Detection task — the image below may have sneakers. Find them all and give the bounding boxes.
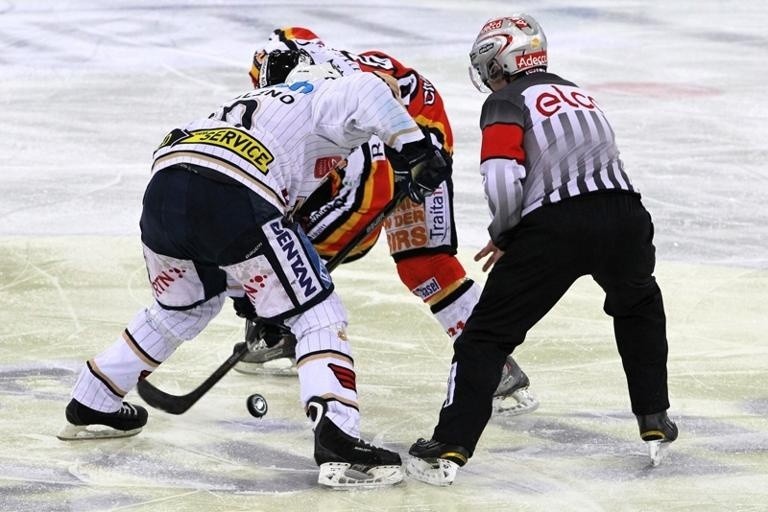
[408,436,471,468]
[233,332,297,364]
[494,355,530,398]
[636,409,679,443]
[313,415,402,473]
[64,398,148,431]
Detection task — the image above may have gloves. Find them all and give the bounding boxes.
[399,138,453,206]
[229,292,258,321]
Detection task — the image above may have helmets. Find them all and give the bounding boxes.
[259,48,311,89]
[467,11,548,93]
[285,61,344,84]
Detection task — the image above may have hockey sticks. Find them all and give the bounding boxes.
[137,185,410,413]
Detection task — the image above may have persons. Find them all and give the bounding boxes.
[235,27,529,397]
[66,52,450,469]
[403,12,677,468]
